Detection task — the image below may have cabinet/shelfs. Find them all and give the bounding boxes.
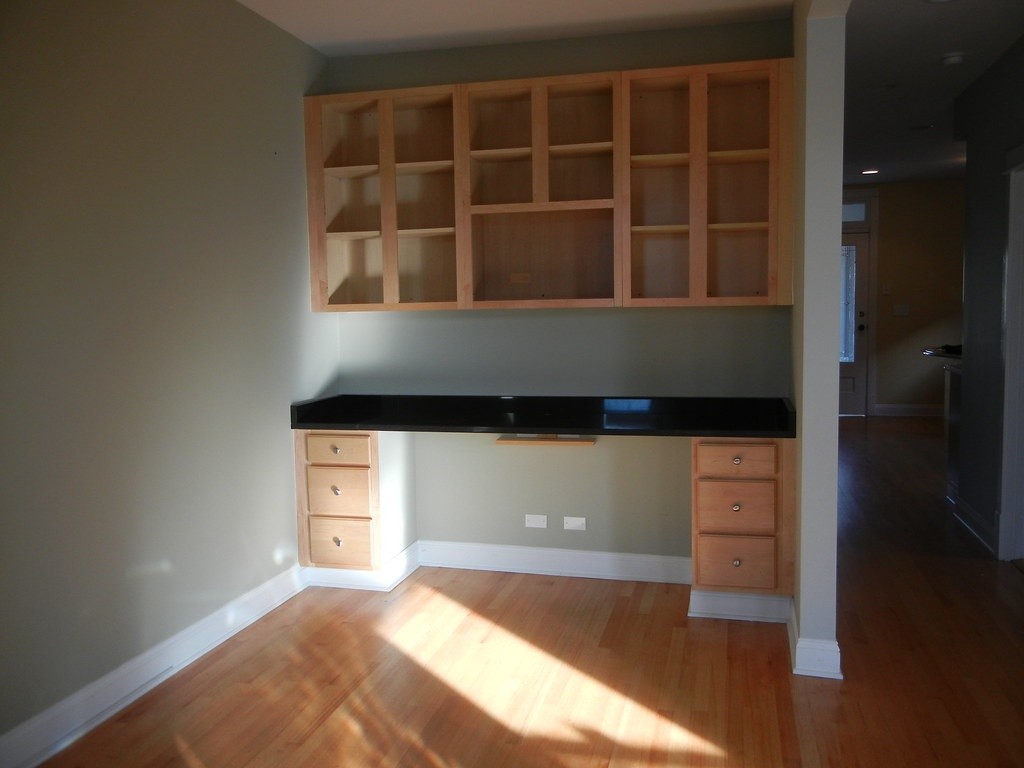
[303,61,793,313]
[293,397,794,620]
